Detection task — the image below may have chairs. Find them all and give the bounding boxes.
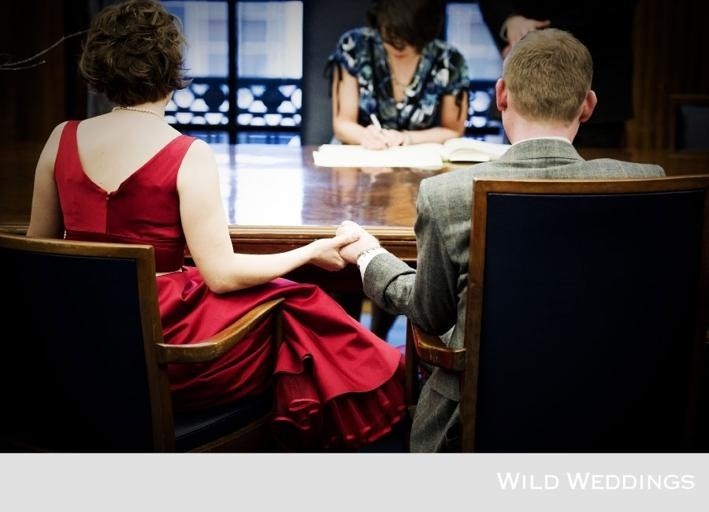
[403,173,708,453]
[0,232,285,453]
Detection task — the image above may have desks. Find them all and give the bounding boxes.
[0,139,709,263]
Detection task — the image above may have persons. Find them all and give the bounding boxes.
[24,0,670,453]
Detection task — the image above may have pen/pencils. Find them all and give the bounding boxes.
[369,113,390,149]
